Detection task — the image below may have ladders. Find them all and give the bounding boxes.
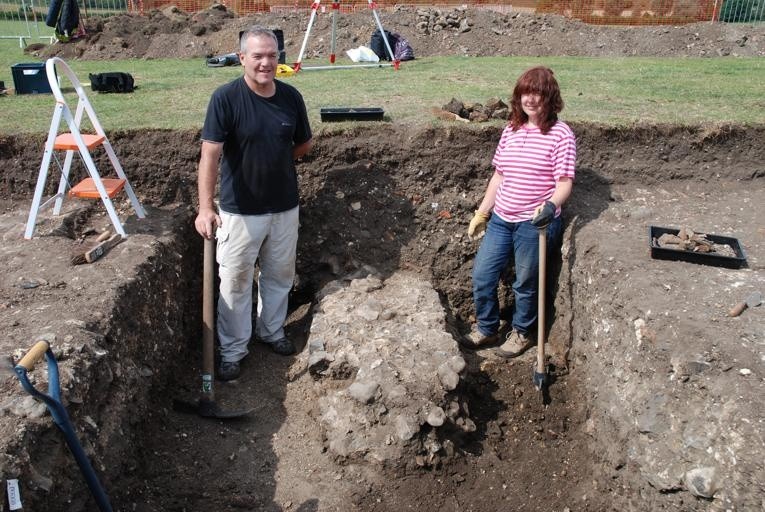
[24,57,147,241]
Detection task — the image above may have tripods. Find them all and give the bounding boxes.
[290,0,401,75]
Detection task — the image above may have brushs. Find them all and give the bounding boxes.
[70,233,121,266]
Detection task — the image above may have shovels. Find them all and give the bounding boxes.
[730,292,762,317]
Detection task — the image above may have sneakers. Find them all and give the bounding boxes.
[499,327,534,358]
[217,361,241,380]
[462,329,498,350]
[270,337,295,355]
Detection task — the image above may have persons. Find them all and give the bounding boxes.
[460,67,576,355]
[193,27,313,381]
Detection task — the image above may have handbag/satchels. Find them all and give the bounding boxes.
[395,37,413,61]
[89,73,134,93]
[371,30,396,60]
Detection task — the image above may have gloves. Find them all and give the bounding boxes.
[468,209,490,242]
[531,201,556,229]
